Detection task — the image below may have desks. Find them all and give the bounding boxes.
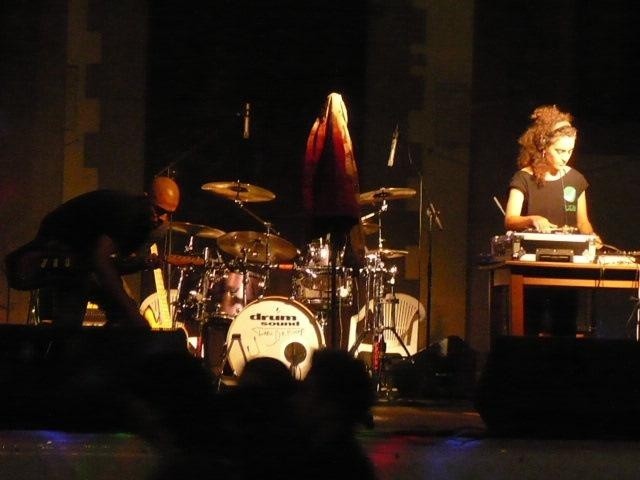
[479,261,640,355]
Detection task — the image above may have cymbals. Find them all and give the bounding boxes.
[165,222,226,240]
[366,249,408,262]
[165,254,204,267]
[201,179,277,203]
[218,231,296,263]
[357,222,380,234]
[356,186,416,205]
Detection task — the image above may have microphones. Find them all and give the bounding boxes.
[386,124,399,168]
[241,102,252,141]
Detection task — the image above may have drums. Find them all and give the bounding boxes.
[226,294,325,385]
[292,266,354,310]
[200,262,271,330]
[294,233,347,275]
[173,264,210,325]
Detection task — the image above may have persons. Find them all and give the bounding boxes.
[503,102,605,339]
[34,175,182,331]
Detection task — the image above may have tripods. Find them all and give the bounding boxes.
[345,325,416,396]
